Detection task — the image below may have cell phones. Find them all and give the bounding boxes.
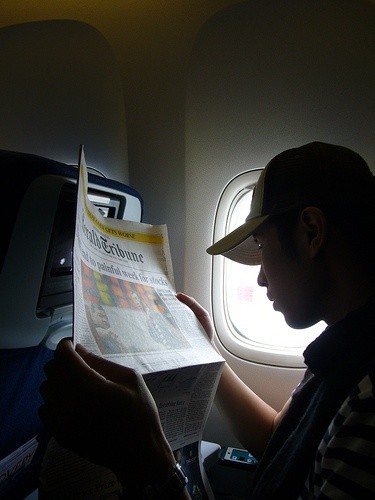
[223,446,258,468]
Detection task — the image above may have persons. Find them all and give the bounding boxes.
[37,141,375,500]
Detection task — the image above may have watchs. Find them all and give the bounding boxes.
[115,461,189,498]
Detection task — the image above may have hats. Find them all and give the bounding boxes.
[207,141,375,277]
